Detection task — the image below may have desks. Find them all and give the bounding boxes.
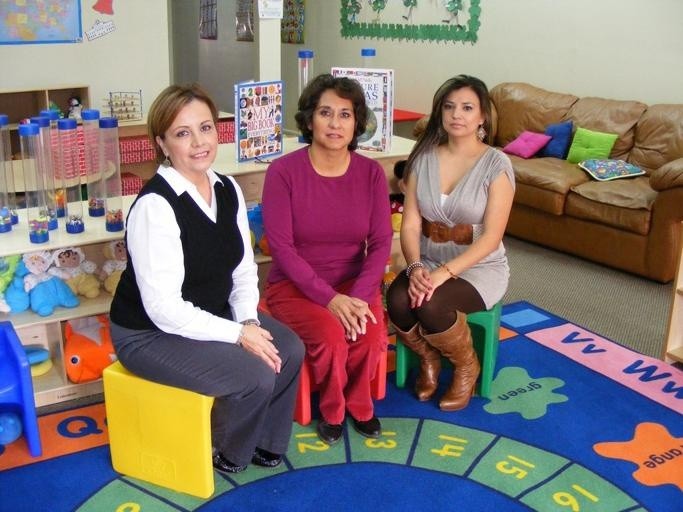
[97,109,236,136]
[393,106,427,129]
[1,158,117,195]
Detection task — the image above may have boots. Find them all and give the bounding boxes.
[391,310,481,411]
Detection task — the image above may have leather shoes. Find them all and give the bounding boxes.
[318,412,343,444]
[213,447,281,473]
[352,415,381,438]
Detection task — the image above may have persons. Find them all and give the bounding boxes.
[109,83,305,474]
[382,74,517,414]
[260,74,394,444]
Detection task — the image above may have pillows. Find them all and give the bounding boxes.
[542,120,571,158]
[503,131,551,159]
[577,157,646,182]
[567,127,617,167]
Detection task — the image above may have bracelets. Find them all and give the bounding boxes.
[405,262,423,277]
[440,264,458,280]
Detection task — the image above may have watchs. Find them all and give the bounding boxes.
[238,318,260,326]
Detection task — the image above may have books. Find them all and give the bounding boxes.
[231,77,285,164]
[326,66,396,156]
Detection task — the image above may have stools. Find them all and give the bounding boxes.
[1,321,42,457]
[101,361,220,498]
[101,289,513,500]
[396,301,501,398]
[258,296,386,425]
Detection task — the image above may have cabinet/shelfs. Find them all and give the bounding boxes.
[193,157,417,334]
[0,194,140,408]
[0,85,88,157]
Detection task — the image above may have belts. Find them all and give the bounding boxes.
[421,219,485,244]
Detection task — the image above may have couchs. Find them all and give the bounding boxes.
[413,83,683,283]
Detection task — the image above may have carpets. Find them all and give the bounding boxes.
[0,301,682,511]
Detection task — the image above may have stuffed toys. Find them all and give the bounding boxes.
[60,312,115,384]
[0,241,130,319]
[386,161,411,231]
